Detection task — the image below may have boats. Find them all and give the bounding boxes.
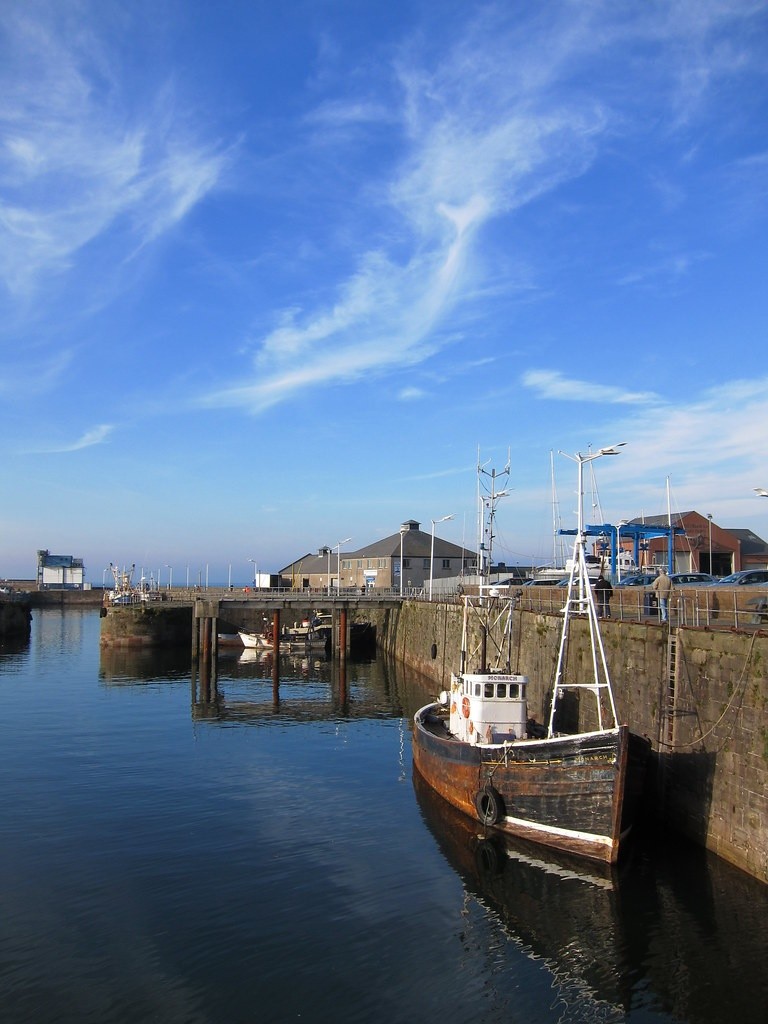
[212,629,245,647]
[98,560,150,613]
[236,607,337,651]
[0,586,33,635]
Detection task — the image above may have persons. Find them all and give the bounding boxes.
[361,584,366,595]
[652,568,675,623]
[353,583,358,591]
[594,575,612,619]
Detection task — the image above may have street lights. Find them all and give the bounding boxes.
[559,440,627,587]
[706,513,717,579]
[248,558,257,588]
[165,564,173,589]
[329,537,352,597]
[479,489,516,606]
[398,522,411,598]
[422,513,459,601]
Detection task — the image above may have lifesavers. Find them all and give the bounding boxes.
[462,697,470,718]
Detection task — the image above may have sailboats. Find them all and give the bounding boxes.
[413,438,630,865]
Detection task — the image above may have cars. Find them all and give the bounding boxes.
[565,574,601,588]
[648,574,717,598]
[555,577,579,589]
[709,567,768,590]
[495,577,532,588]
[617,575,661,589]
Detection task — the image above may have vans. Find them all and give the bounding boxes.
[521,579,561,589]
